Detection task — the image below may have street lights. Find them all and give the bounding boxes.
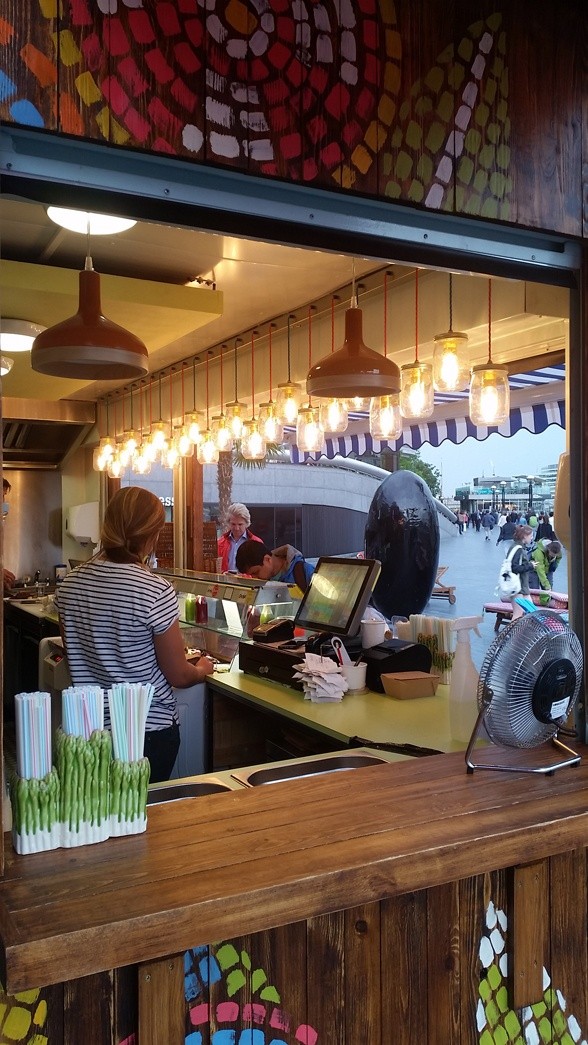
[500,481,507,509]
[526,475,535,507]
[490,485,496,510]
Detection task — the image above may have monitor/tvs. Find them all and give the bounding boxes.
[292,555,383,639]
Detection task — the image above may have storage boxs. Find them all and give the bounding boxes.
[381,671,441,700]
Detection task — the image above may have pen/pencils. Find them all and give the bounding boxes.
[354,653,365,666]
[336,642,343,665]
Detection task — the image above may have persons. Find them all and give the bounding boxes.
[217,503,263,574]
[235,540,315,594]
[506,525,540,621]
[53,487,213,784]
[3,479,12,526]
[454,506,555,555]
[527,537,563,591]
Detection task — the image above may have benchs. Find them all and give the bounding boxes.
[483,602,569,631]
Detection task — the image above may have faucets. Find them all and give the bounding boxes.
[33,569,41,583]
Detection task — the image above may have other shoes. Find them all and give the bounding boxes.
[485,536,491,542]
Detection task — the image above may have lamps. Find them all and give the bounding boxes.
[0,205,510,478]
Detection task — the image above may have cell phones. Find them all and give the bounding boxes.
[278,640,305,649]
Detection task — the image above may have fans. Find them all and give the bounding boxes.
[465,610,583,777]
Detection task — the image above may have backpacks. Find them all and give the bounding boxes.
[525,539,546,563]
[498,545,524,602]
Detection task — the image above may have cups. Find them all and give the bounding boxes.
[339,661,367,689]
[396,623,411,641]
[361,620,386,648]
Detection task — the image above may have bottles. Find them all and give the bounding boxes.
[184,593,196,622]
[146,559,150,568]
[150,555,156,568]
[260,605,274,625]
[55,565,66,582]
[195,595,208,624]
[247,605,262,638]
